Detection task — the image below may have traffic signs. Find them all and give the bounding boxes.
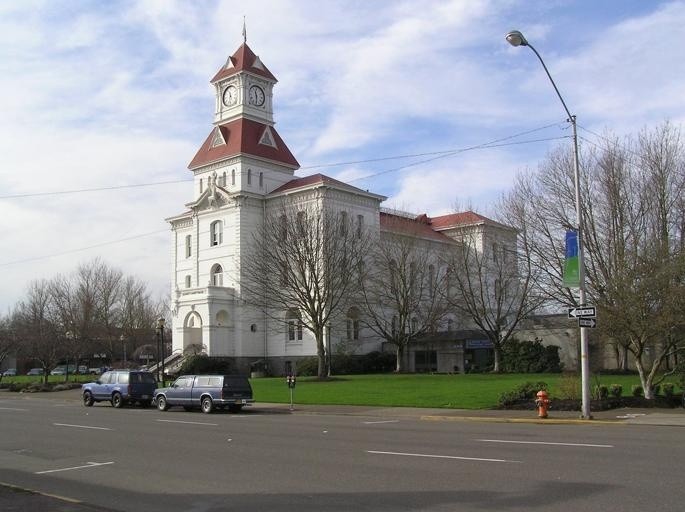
[567,306,596,320]
[579,317,597,329]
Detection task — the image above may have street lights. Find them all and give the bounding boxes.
[506,29,590,420]
[155,315,165,389]
[120,335,125,368]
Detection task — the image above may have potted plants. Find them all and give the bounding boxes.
[250,359,265,378]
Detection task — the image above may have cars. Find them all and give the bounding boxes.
[28,364,100,376]
[3,369,17,376]
[153,375,255,414]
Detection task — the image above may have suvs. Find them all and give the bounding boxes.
[82,369,158,409]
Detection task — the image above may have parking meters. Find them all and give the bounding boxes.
[286,375,296,407]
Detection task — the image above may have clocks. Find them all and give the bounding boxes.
[249,85,265,106]
[223,85,238,107]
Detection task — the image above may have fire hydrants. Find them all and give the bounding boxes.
[536,390,552,418]
